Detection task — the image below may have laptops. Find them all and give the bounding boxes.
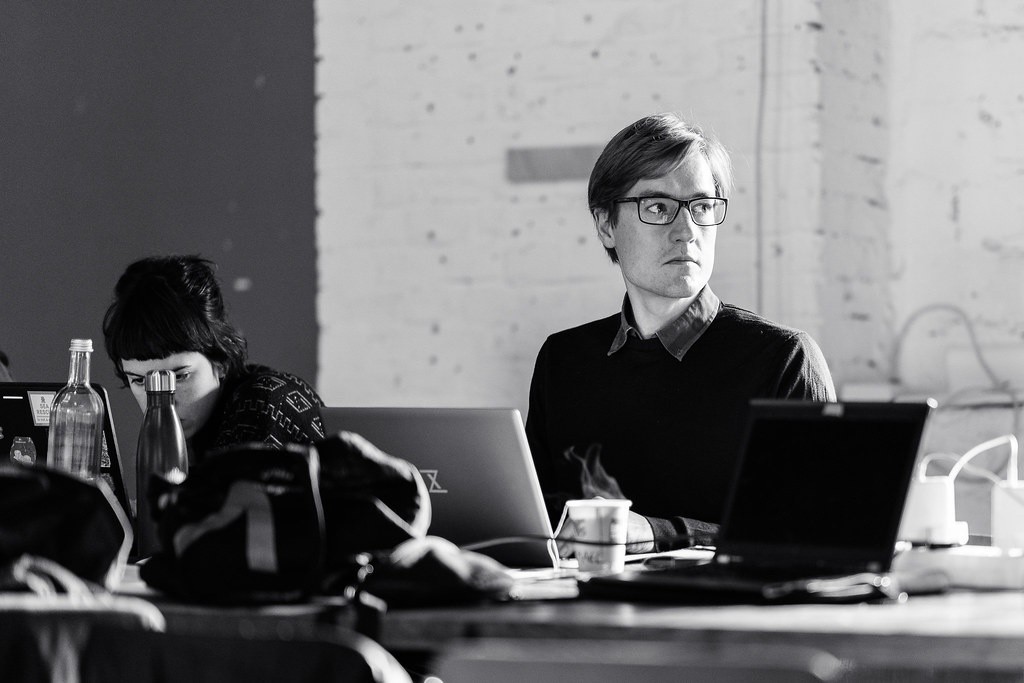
[0,381,133,526]
[624,400,936,581]
[320,406,714,572]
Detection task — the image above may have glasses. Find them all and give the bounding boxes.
[614,196,728,226]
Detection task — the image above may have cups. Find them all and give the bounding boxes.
[566,500,633,576]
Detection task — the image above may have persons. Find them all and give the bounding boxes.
[102,256,330,610]
[526,113,839,556]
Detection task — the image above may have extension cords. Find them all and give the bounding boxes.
[891,545,1024,592]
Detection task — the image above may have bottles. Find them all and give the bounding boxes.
[47,339,104,485]
[136,370,189,556]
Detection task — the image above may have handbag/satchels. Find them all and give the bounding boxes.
[143,429,432,605]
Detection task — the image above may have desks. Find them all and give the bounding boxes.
[364,585,1024,683]
